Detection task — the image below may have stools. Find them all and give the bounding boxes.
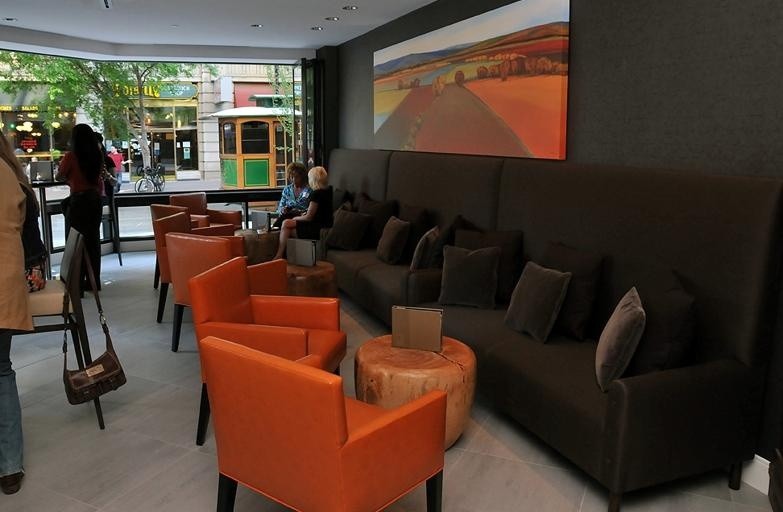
[152,211,245,322]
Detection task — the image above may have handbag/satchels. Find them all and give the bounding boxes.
[63,232,127,404]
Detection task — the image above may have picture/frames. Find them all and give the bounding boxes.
[372,0,572,160]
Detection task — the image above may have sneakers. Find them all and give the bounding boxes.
[0,470,23,494]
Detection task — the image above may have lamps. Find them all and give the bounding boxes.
[341,4,358,14]
[323,16,340,23]
[3,16,17,24]
[310,26,323,33]
[250,23,264,28]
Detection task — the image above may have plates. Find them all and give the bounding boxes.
[272,226,278,229]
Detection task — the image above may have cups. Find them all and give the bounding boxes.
[303,191,309,197]
[257,229,265,235]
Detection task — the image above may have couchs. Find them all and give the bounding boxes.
[316,147,778,512]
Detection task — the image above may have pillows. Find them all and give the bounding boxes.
[325,210,373,251]
[402,205,430,224]
[595,284,646,394]
[629,260,702,369]
[448,216,525,306]
[408,222,442,275]
[374,216,412,266]
[501,261,573,344]
[539,242,604,335]
[353,191,398,219]
[330,188,348,208]
[437,243,502,310]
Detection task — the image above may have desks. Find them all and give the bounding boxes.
[29,177,124,280]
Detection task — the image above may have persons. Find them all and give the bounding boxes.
[60,132,118,217]
[271,165,333,261]
[0,156,35,496]
[107,146,133,193]
[272,163,313,230]
[55,124,105,298]
[0,129,48,293]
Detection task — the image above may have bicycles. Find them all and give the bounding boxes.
[135,168,165,192]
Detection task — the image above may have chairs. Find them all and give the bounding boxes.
[12,228,106,430]
[169,188,242,227]
[186,256,347,445]
[149,199,235,289]
[164,230,287,352]
[196,334,449,512]
